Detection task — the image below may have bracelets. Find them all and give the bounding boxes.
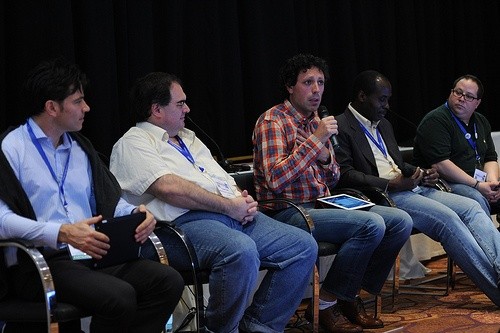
[473,180,479,189]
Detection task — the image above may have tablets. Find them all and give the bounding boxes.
[317,194,375,210]
[92,213,145,270]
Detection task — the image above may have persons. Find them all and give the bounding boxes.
[108,70,318,333]
[413,74,500,232]
[0,71,186,333]
[332,68,500,308]
[251,53,413,332]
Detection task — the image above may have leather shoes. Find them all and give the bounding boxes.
[338,296,384,329]
[304,301,363,333]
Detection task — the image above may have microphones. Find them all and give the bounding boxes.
[400,166,426,177]
[186,116,249,173]
[318,105,337,146]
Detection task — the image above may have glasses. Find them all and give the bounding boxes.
[451,89,477,102]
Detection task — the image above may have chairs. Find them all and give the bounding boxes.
[0,157,458,333]
